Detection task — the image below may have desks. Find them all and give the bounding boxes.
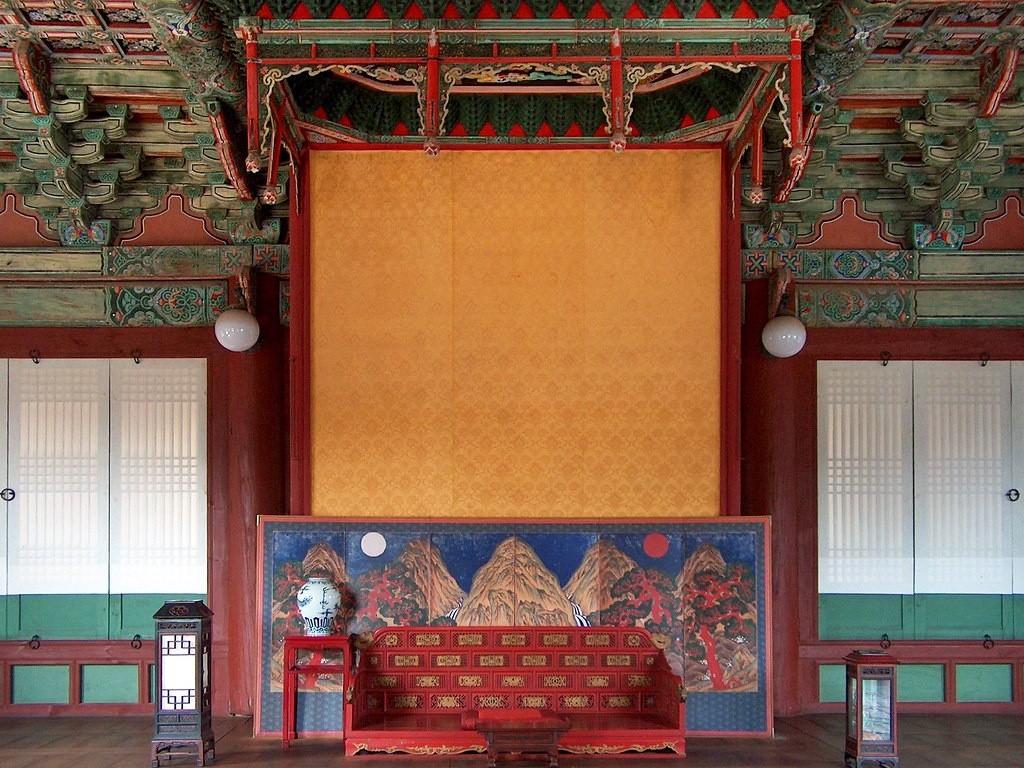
[282,634,355,750]
[474,717,573,768]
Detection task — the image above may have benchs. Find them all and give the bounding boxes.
[345,626,687,758]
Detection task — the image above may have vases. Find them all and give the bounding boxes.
[297,577,342,636]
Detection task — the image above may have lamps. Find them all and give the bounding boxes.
[761,294,807,359]
[214,267,260,353]
